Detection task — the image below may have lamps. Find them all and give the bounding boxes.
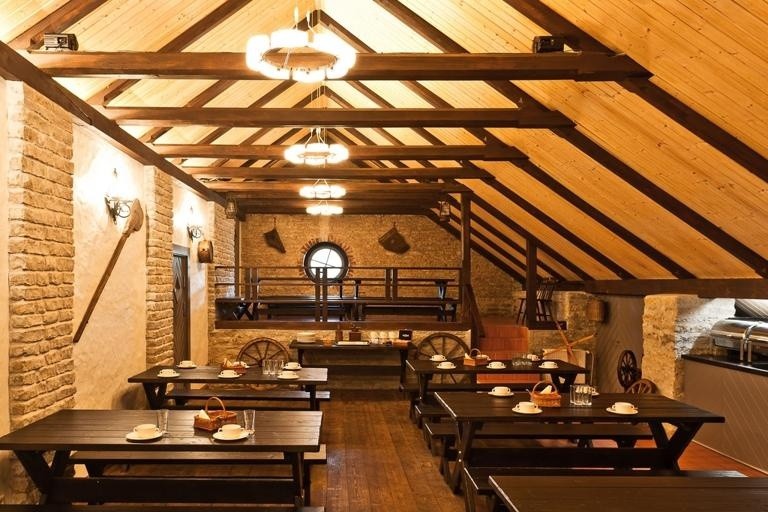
[104,168,142,224]
[222,189,239,222]
[186,206,206,241]
[247,0,358,217]
[438,189,450,224]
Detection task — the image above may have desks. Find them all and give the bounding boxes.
[287,338,415,383]
[215,294,254,320]
[435,392,726,509]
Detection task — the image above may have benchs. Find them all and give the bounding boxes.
[0,498,327,512]
[164,388,330,411]
[255,275,457,321]
[397,381,751,511]
[67,443,328,504]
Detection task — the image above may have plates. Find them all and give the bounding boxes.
[176,364,198,369]
[211,431,250,441]
[157,374,179,379]
[284,367,302,371]
[124,431,162,441]
[295,333,318,346]
[217,375,239,379]
[278,376,299,380]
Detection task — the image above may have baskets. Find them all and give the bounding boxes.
[529,381,561,407]
[221,354,247,375]
[193,397,237,431]
[462,349,489,366]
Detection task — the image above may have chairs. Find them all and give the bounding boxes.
[515,277,560,324]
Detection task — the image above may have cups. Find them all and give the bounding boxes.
[262,359,284,376]
[220,370,237,377]
[243,410,256,436]
[156,409,169,436]
[133,424,157,436]
[280,371,296,378]
[285,362,300,368]
[426,353,638,415]
[160,369,176,375]
[179,360,193,365]
[217,422,242,439]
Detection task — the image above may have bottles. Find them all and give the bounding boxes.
[334,322,343,341]
